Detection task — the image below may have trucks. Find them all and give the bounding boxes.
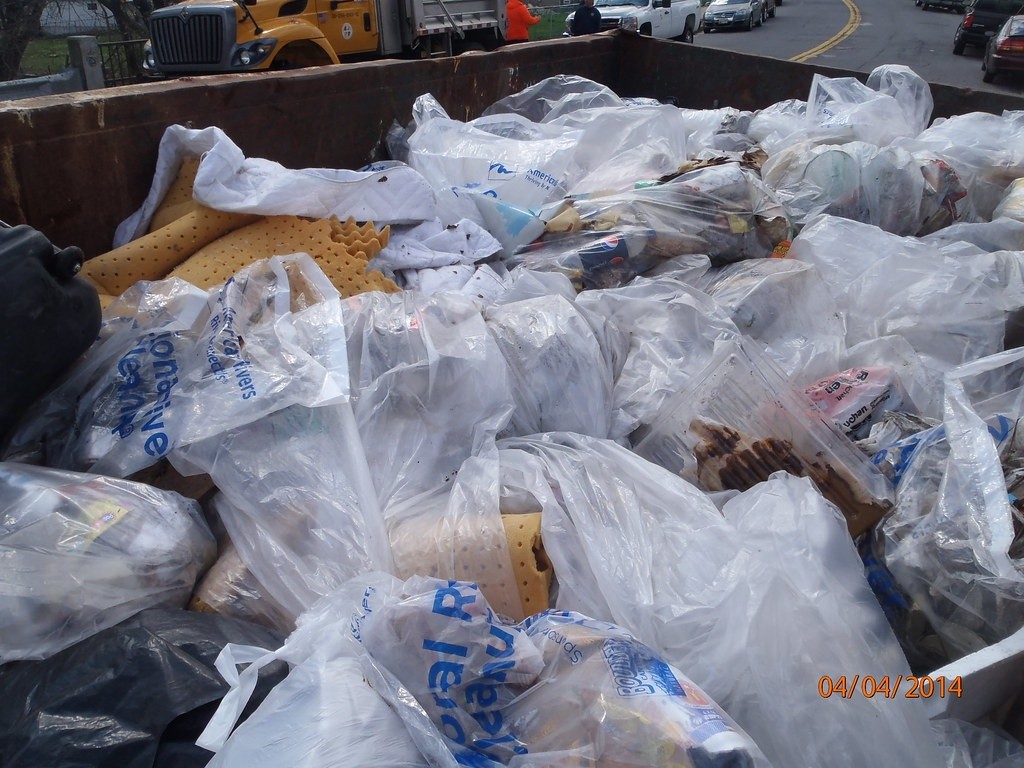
[140,0,505,80]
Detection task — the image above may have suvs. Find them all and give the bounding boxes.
[563,0,701,44]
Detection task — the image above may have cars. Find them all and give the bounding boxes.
[703,0,782,33]
[915,0,1024,83]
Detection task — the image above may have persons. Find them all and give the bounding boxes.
[570,0,601,37]
[505,0,542,45]
